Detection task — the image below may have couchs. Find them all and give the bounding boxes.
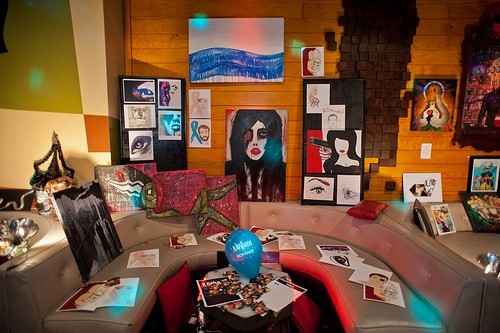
[0,189,500,332]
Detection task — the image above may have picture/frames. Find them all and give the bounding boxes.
[222,108,286,203]
[300,77,366,207]
[117,75,187,172]
[410,76,459,132]
[464,155,500,194]
[188,119,212,150]
[93,161,155,212]
[188,90,212,120]
[187,15,286,84]
[449,23,500,149]
[299,46,325,78]
[403,171,445,204]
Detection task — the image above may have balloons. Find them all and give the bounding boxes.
[224,228,264,286]
